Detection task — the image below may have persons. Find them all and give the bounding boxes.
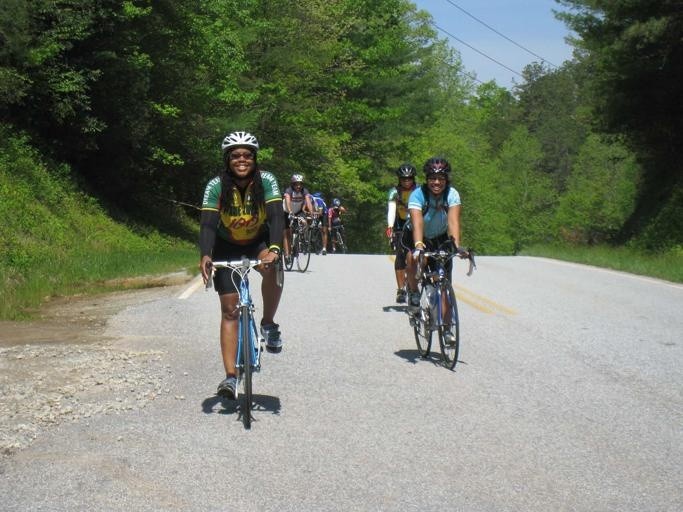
[398,159,462,348]
[328,199,346,254]
[302,194,328,255]
[200,130,287,400]
[282,174,313,264]
[387,165,417,304]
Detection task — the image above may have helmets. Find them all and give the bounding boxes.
[422,158,450,176]
[399,165,415,178]
[291,175,304,183]
[221,132,259,150]
[332,198,340,205]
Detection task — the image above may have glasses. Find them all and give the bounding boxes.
[227,152,254,160]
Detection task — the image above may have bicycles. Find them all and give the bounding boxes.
[406,237,476,368]
[388,229,426,302]
[282,213,320,272]
[204,251,284,430]
[303,218,347,254]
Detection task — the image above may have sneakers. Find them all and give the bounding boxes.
[218,376,235,400]
[397,288,405,302]
[409,292,420,314]
[444,331,455,343]
[260,320,282,353]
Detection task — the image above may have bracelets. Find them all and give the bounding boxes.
[415,241,424,247]
[269,244,280,252]
